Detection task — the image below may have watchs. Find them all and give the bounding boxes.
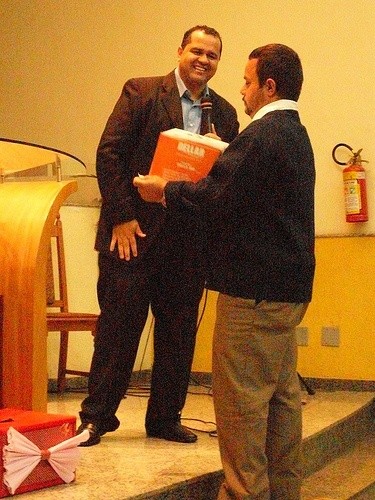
[160,189,167,208]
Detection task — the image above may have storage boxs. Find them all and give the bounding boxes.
[147,128,230,183]
[0,408,90,499]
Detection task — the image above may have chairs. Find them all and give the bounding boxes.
[46,213,100,395]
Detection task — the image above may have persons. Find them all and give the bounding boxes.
[132,41,317,500]
[76,23,239,448]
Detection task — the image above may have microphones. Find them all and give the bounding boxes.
[199,97,212,136]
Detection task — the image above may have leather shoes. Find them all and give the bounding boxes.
[76,414,120,446]
[145,420,197,442]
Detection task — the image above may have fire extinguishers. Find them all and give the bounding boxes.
[332,143,369,222]
